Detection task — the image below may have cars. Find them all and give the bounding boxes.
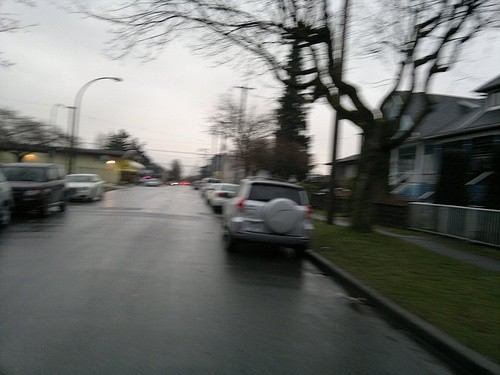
[0,175,15,228]
[66,173,105,205]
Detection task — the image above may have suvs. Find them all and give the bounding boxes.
[193,178,240,212]
[221,177,315,260]
[0,162,66,222]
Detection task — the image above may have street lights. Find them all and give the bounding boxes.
[67,76,122,175]
